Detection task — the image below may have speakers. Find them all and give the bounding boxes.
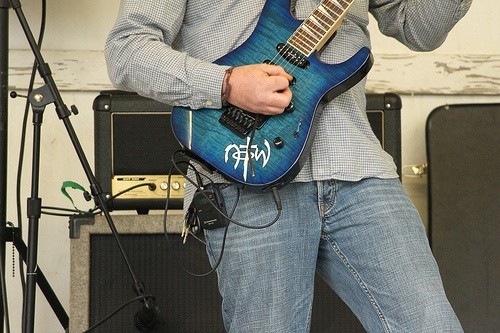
[67,214,365,333]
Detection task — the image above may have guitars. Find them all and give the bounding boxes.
[170,0,374,192]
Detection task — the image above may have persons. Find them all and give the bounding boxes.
[105,1,473,333]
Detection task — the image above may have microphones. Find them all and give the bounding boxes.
[132,298,160,333]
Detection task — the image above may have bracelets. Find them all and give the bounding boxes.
[221,66,236,107]
